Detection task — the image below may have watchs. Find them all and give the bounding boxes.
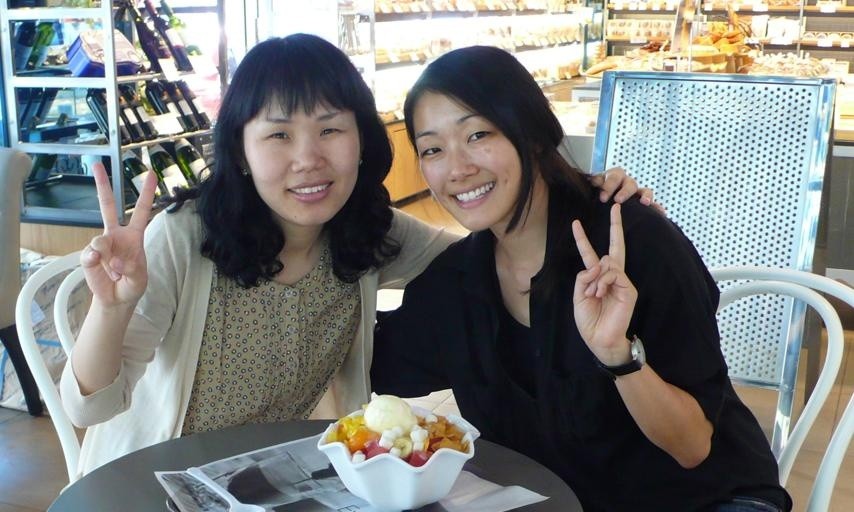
[592,331,648,379]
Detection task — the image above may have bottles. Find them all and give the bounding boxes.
[146,142,190,199]
[10,2,195,76]
[13,85,62,129]
[177,139,212,185]
[87,77,207,145]
[123,150,163,207]
[26,114,67,186]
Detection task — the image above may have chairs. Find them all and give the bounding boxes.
[702,265,853,512]
[588,68,836,467]
[14,249,94,486]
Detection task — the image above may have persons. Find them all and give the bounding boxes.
[53,32,667,482]
[367,45,795,512]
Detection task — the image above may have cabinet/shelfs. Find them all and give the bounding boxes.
[0,0,215,416]
[606,1,854,75]
[335,2,590,210]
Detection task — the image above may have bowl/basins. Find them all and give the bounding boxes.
[317,405,481,510]
